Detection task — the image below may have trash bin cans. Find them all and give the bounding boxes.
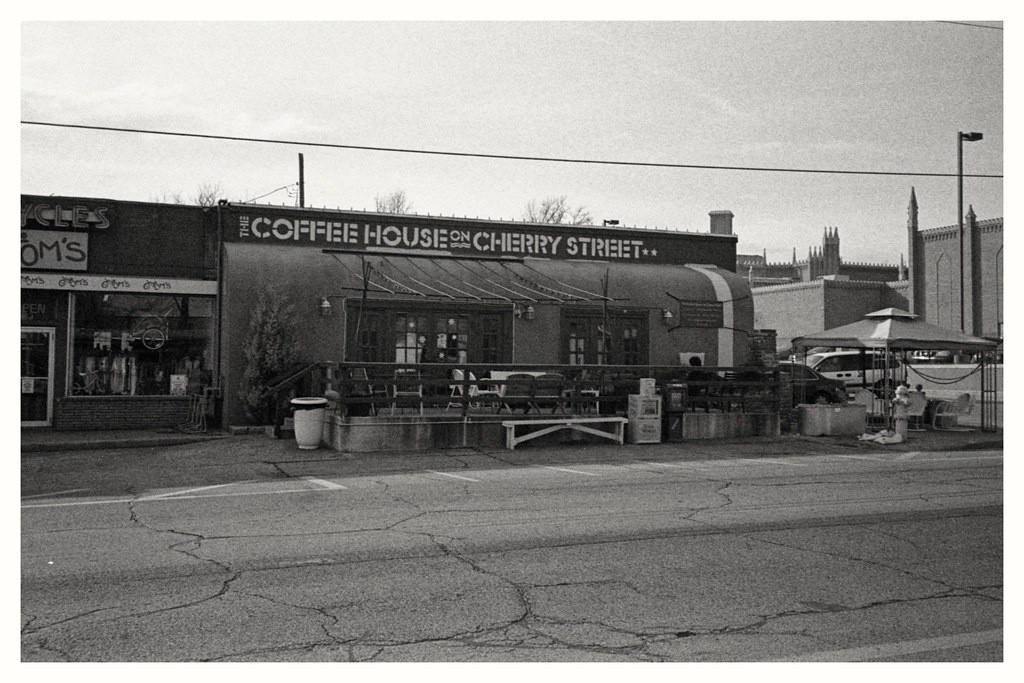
[291,397,328,449]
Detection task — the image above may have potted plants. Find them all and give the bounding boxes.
[797,389,866,435]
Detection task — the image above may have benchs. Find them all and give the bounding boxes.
[502,417,628,449]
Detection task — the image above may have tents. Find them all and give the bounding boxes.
[794,308,997,430]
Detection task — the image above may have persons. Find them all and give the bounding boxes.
[687,356,722,381]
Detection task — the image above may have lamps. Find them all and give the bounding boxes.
[663,309,673,325]
[525,305,534,319]
[321,297,332,316]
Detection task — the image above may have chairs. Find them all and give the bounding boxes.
[854,389,928,431]
[336,369,775,415]
[932,393,974,431]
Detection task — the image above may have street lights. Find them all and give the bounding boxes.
[957,130,985,332]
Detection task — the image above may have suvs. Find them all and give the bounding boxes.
[780,346,911,405]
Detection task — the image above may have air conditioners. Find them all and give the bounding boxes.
[679,352,705,367]
